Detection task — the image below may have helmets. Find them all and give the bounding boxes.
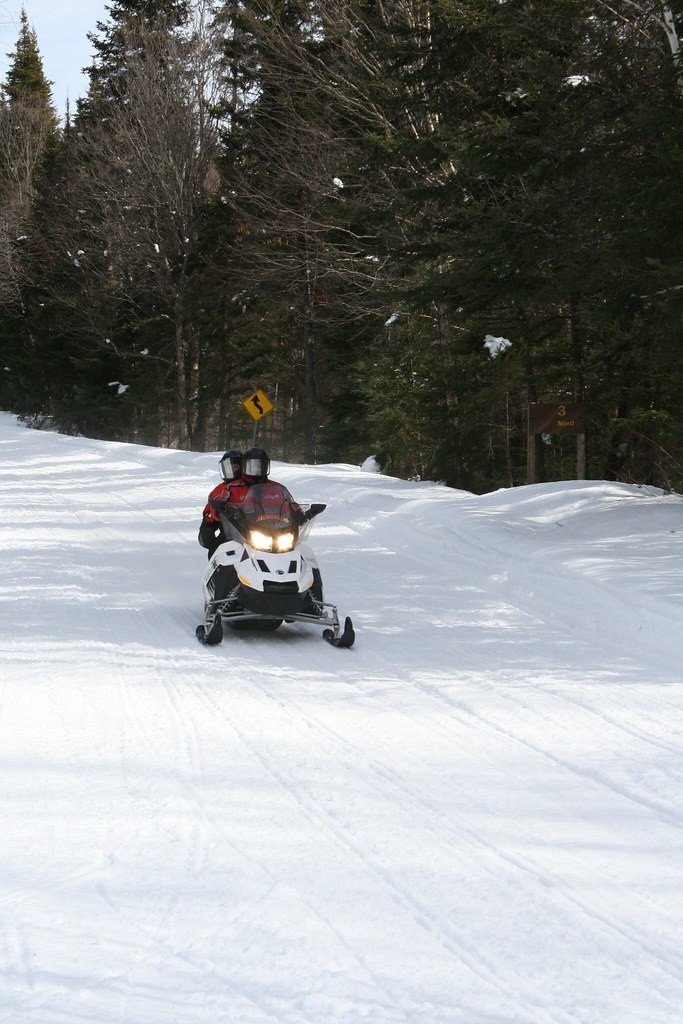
[218,449,244,481]
[242,447,270,479]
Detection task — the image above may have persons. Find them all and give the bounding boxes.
[198,450,245,550]
[211,448,307,555]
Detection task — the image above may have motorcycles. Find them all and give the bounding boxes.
[195,483,356,649]
